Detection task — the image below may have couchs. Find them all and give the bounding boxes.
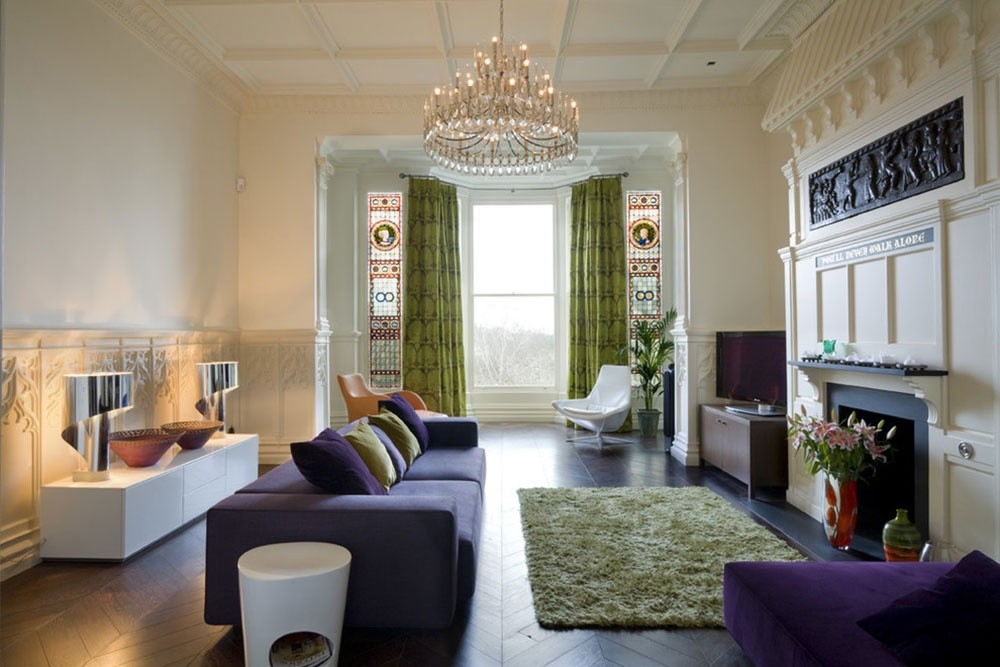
[205,417,485,629]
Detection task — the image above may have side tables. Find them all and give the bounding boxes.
[237,541,352,667]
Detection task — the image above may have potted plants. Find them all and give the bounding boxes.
[617,306,678,438]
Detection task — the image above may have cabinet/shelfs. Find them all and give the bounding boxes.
[39,433,260,563]
[699,403,788,499]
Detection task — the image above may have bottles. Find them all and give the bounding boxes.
[883,509,920,562]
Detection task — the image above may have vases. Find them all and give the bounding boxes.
[883,509,920,561]
[822,468,858,551]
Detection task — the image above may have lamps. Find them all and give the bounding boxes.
[424,0,579,175]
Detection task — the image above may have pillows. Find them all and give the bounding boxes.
[367,405,421,470]
[857,550,1000,667]
[378,392,430,454]
[290,427,388,495]
[369,425,407,483]
[342,420,396,493]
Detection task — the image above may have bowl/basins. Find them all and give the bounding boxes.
[110,428,185,467]
[162,421,224,449]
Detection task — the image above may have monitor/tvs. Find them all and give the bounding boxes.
[716,331,788,416]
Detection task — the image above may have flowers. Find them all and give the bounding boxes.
[786,405,898,487]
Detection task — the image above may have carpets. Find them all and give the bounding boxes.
[517,486,808,628]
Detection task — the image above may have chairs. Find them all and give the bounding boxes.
[552,365,635,449]
[724,561,957,667]
[338,374,448,423]
